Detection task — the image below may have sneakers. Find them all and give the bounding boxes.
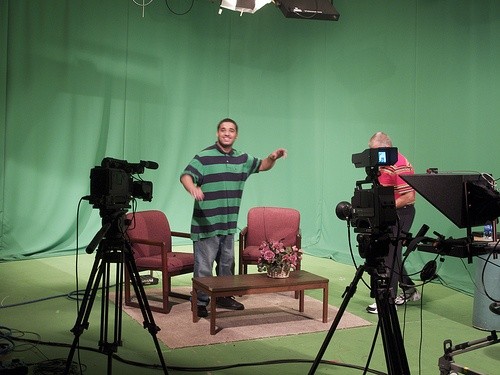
[394,289,421,304]
[366,302,397,313]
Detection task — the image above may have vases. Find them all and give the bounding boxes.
[266,265,290,278]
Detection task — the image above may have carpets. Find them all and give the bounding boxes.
[103,285,374,350]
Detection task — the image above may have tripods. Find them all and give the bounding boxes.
[66,210,170,375]
[308,225,411,375]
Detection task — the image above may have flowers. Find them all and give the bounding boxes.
[257,238,306,277]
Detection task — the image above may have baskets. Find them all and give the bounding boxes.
[267,262,290,279]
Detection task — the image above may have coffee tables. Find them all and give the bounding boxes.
[192,270,330,336]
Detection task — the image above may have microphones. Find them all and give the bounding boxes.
[140,160,159,169]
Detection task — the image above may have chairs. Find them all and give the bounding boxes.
[125,210,195,314]
[239,207,301,299]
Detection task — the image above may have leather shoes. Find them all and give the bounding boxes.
[217,296,244,310]
[191,302,208,317]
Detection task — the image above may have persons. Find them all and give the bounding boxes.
[180,118,286,317]
[366,132,421,313]
